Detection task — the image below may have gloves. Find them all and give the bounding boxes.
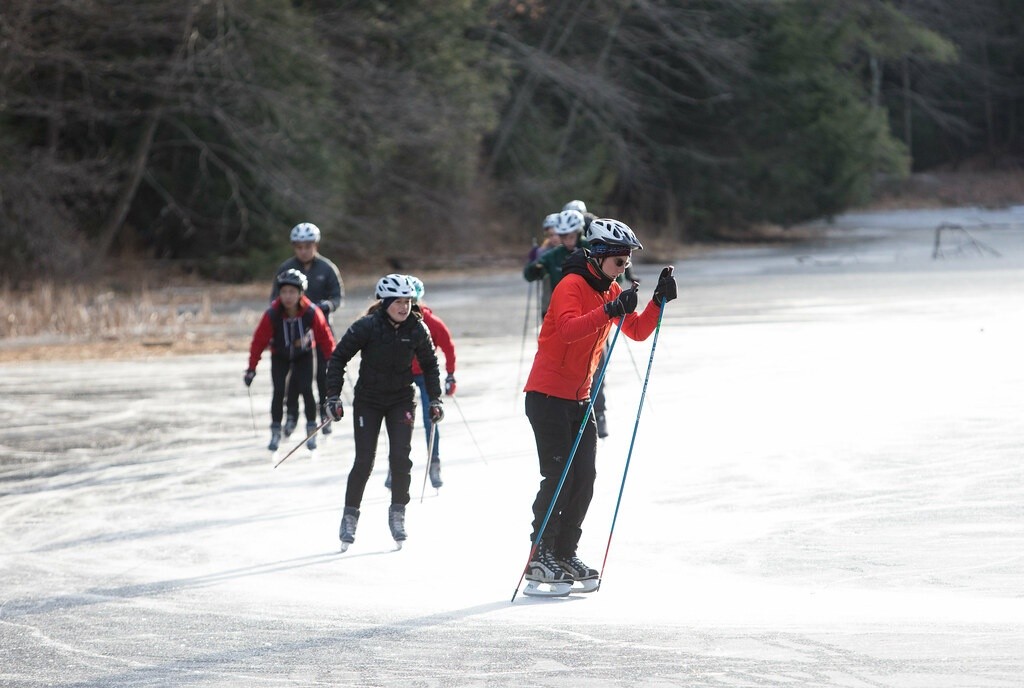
[605,289,637,318]
[244,370,256,386]
[325,397,344,422]
[429,400,444,423]
[652,268,678,307]
[445,374,456,397]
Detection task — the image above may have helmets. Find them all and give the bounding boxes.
[289,222,321,243]
[376,274,416,298]
[275,268,308,290]
[563,200,587,215]
[585,217,643,250]
[543,213,560,229]
[553,210,585,233]
[408,276,425,303]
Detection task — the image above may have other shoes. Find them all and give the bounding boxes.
[597,415,608,438]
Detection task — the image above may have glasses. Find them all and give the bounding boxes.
[615,258,631,269]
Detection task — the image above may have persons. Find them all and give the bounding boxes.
[245,269,336,452]
[523,218,678,598]
[384,277,456,496]
[270,222,343,439]
[524,200,641,441]
[326,274,444,551]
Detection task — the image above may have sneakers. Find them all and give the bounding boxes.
[339,507,360,551]
[429,458,443,495]
[267,429,282,455]
[321,415,331,437]
[306,423,319,454]
[284,415,297,439]
[523,538,574,597]
[385,469,392,488]
[556,553,600,592]
[389,503,407,549]
